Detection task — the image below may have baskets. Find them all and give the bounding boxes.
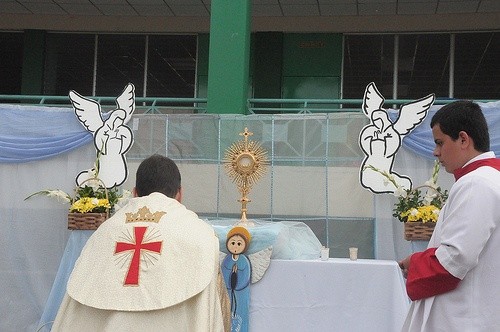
[67,178,111,230]
[404,185,444,241]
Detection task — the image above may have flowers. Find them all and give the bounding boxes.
[363,160,449,224]
[23,185,132,214]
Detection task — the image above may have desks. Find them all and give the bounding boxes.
[35,216,321,332]
[249,257,408,332]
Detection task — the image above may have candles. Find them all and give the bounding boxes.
[349,247,358,261]
[321,245,330,261]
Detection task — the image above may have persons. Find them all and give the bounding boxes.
[391,102,500,332]
[50,153,231,332]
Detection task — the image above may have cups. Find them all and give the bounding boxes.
[349,247,358,261]
[321,247,330,261]
